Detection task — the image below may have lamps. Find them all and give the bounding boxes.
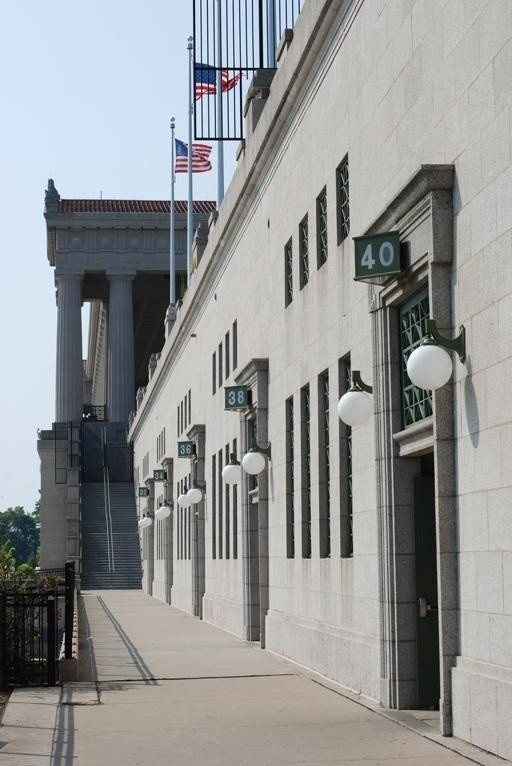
[405,318,465,391]
[336,369,374,429]
[241,436,271,476]
[154,499,174,521]
[138,512,154,529]
[221,453,242,485]
[177,479,206,508]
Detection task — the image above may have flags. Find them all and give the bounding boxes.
[174,137,214,175]
[193,61,244,103]
